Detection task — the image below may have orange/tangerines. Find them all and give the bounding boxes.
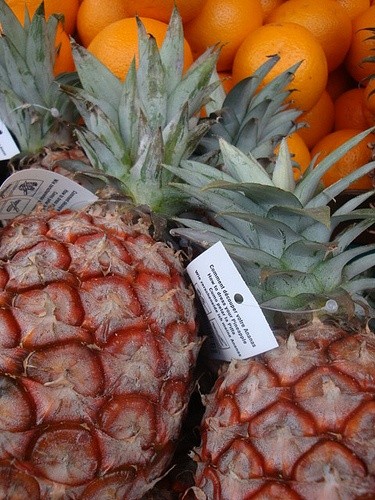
[0,0,375,189]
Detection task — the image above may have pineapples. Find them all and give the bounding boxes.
[160,124,375,500]
[0,26,309,500]
